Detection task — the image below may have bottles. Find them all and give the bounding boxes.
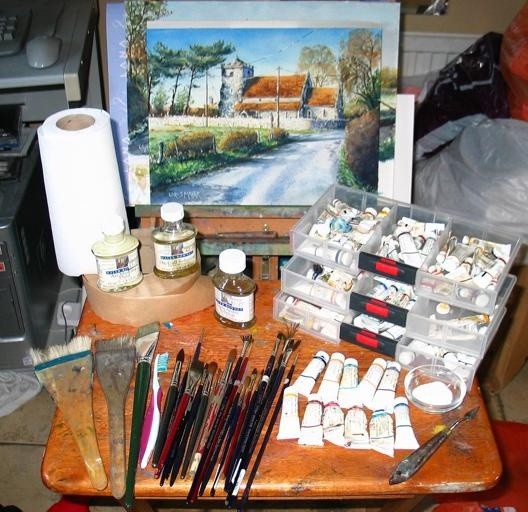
[151,202,200,280]
[212,248,258,330]
[92,215,145,293]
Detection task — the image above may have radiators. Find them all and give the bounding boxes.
[398,31,485,79]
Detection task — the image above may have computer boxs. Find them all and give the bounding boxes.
[0,126,62,371]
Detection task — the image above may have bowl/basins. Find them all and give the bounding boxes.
[403,365,467,415]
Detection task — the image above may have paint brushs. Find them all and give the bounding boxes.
[124,320,300,509]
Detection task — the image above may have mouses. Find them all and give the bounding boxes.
[27,34,61,69]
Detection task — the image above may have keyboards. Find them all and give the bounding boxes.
[0,0,32,55]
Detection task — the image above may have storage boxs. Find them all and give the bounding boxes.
[479,261,527,398]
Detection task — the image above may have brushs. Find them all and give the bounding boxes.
[27,323,136,500]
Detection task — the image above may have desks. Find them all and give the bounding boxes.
[41,280,504,512]
[0,0,102,125]
